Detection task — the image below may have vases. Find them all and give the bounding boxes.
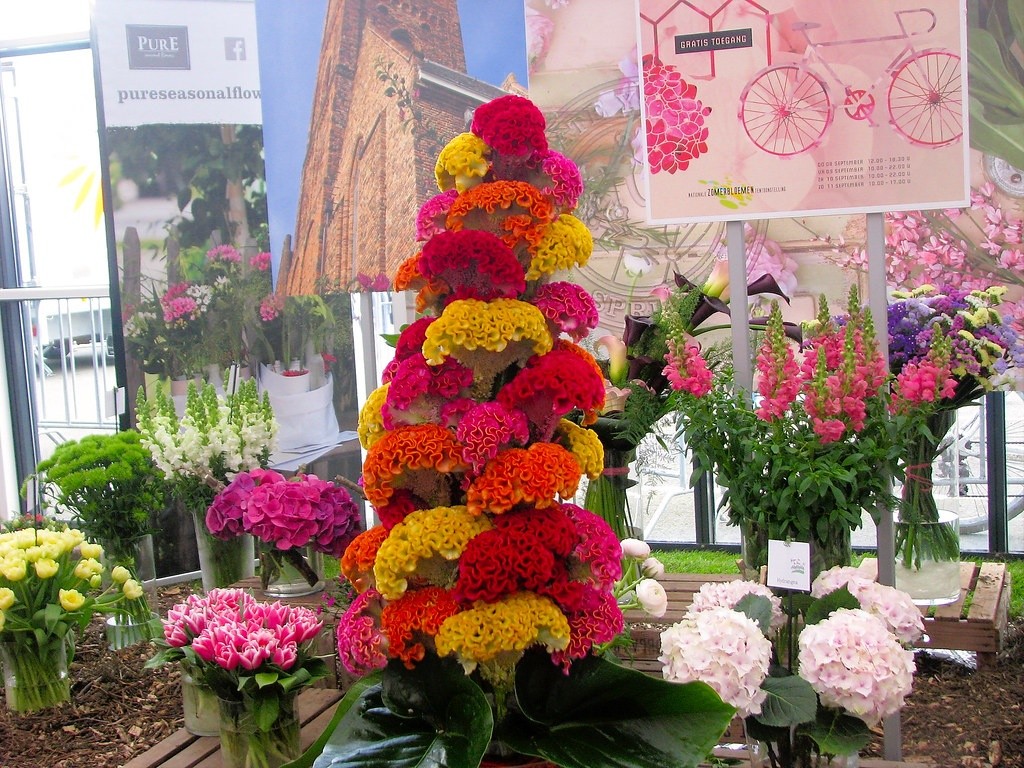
[97,534,162,651]
[1,635,71,712]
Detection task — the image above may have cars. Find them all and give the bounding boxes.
[31,298,114,360]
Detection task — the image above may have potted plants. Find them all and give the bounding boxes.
[19,427,164,651]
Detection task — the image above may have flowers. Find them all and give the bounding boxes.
[0,526,145,713]
[129,55,1024,768]
